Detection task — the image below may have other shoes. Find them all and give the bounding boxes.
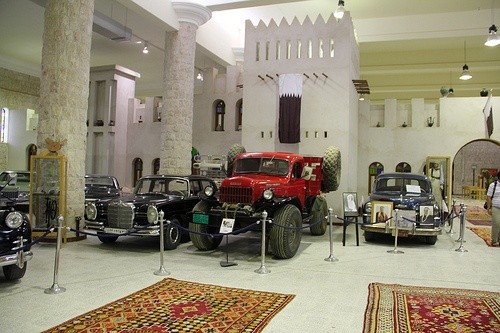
[491,242,496,247]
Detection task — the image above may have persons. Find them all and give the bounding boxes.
[345,194,355,211]
[421,207,433,223]
[429,161,443,189]
[376,206,388,222]
[487,171,500,245]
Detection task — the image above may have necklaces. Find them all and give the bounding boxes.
[378,212,385,220]
[421,214,429,222]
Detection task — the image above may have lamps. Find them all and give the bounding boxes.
[334,0,346,22]
[197,68,202,79]
[459,39,474,80]
[142,40,148,53]
[358,92,365,101]
[483,2,500,47]
[440,70,454,97]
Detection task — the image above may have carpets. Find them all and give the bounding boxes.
[452,205,500,246]
[362,282,500,333]
[41,278,296,333]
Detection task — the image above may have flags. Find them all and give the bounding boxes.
[483,93,494,138]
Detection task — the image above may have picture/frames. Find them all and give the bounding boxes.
[342,192,359,218]
[418,205,435,227]
[371,201,393,226]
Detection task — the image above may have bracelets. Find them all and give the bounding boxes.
[489,208,491,209]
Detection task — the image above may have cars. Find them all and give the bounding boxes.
[85,174,123,199]
[0,171,36,281]
[360,173,441,245]
[84,174,218,251]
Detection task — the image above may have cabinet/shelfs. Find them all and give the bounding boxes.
[28,156,67,247]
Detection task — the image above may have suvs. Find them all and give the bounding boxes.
[186,145,342,260]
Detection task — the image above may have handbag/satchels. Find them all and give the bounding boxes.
[484,202,492,209]
